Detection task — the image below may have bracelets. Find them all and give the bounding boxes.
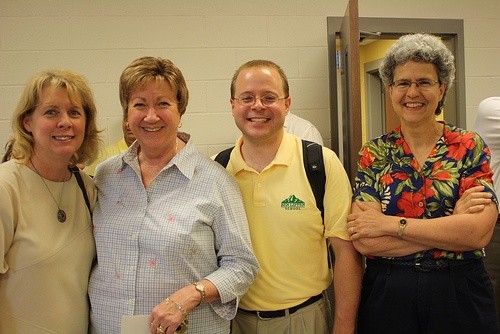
[166,296,189,320]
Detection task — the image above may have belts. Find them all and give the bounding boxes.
[237,291,324,320]
[372,256,480,271]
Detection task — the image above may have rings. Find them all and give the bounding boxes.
[156,326,165,333]
[352,227,354,233]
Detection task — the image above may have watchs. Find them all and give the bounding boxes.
[398,218,407,238]
[194,282,206,308]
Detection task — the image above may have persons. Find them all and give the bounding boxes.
[82,56,260,334]
[0,70,98,334]
[206,59,364,334]
[347,34,500,334]
[474,96,500,204]
[82,113,141,178]
[236,108,323,146]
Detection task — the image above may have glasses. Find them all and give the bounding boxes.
[389,79,440,91]
[232,94,288,107]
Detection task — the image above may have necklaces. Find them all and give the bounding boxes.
[32,155,68,223]
[136,138,178,176]
